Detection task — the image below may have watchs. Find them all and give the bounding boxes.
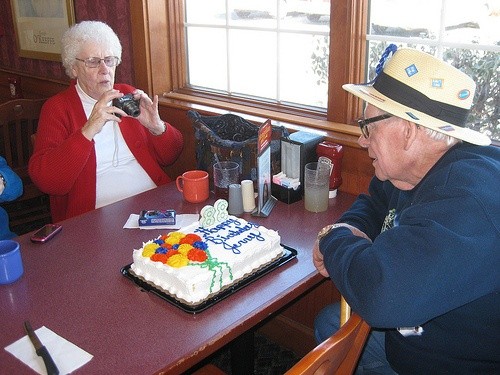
[318,224,337,239]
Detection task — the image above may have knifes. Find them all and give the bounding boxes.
[24,319,60,375]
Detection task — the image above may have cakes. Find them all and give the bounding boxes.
[131,200,283,304]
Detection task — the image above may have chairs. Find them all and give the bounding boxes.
[0,97,52,233]
[281,314,370,375]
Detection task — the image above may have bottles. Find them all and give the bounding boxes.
[241,179,256,212]
[228,183,245,216]
[315,141,344,199]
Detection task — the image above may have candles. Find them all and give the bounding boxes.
[214,199,230,222]
[199,202,214,227]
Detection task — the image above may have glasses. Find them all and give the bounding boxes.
[358,113,394,139]
[75,56,120,68]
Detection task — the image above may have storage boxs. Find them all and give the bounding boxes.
[139,209,176,226]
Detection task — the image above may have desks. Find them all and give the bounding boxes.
[0,178,359,375]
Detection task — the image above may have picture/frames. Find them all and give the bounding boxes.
[10,0,75,63]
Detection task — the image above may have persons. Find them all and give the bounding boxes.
[0,156,24,240]
[313,44,500,375]
[28,22,183,224]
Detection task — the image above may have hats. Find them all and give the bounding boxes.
[342,44,491,146]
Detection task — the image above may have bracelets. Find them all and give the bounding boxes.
[0,176,6,186]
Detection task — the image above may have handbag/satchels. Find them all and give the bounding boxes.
[187,109,290,190]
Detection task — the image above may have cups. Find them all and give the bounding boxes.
[175,170,209,204]
[0,240,25,285]
[213,161,240,201]
[304,162,330,212]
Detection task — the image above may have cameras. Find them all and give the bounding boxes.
[114,94,141,118]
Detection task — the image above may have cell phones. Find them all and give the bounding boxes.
[31,223,62,244]
